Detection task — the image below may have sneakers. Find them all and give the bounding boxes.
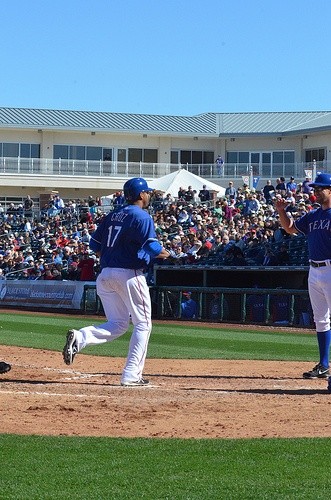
[62,329,80,366]
[302,362,331,393]
[121,379,150,386]
[1,360,13,376]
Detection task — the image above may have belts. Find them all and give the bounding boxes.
[309,260,331,267]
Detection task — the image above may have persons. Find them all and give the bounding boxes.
[273,174,331,392]
[62,178,170,386]
[0,154,322,324]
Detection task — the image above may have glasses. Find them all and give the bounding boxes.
[312,187,328,192]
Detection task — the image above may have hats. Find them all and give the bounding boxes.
[243,184,248,187]
[229,181,233,184]
[163,232,168,237]
[290,176,294,179]
[309,172,331,187]
[250,229,256,233]
[187,232,195,236]
[124,178,156,198]
